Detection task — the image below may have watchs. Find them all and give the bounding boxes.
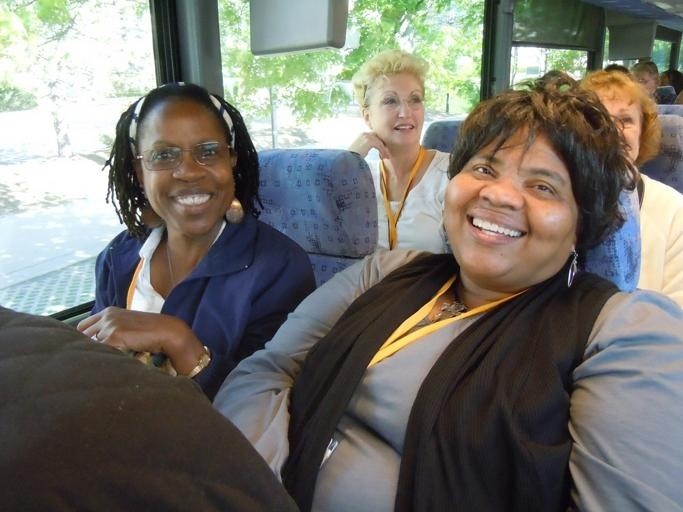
[186,344,211,382]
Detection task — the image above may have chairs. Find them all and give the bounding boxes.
[0,86,683,512]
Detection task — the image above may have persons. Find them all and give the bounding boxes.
[573,67,682,313]
[209,69,682,512]
[603,64,631,76]
[658,69,682,103]
[630,59,676,105]
[73,77,318,407]
[344,49,454,257]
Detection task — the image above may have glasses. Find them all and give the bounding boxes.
[135,141,231,172]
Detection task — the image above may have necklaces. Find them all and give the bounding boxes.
[427,283,468,324]
[163,237,179,289]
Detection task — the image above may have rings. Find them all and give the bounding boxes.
[91,330,102,342]
